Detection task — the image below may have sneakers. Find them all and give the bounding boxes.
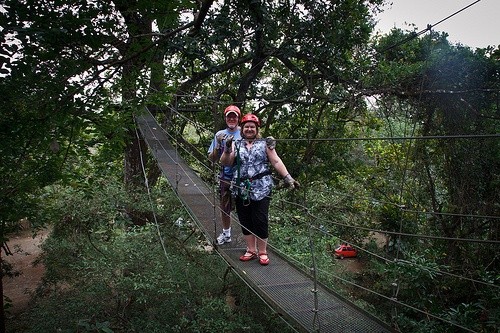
[217,233,231,245]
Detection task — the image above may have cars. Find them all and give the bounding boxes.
[334,243,362,260]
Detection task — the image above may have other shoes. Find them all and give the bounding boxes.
[258,253,270,265]
[240,251,257,260]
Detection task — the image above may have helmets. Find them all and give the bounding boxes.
[241,114,260,126]
[224,106,241,117]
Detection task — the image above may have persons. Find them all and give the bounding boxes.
[207,106,276,246]
[220,115,299,266]
